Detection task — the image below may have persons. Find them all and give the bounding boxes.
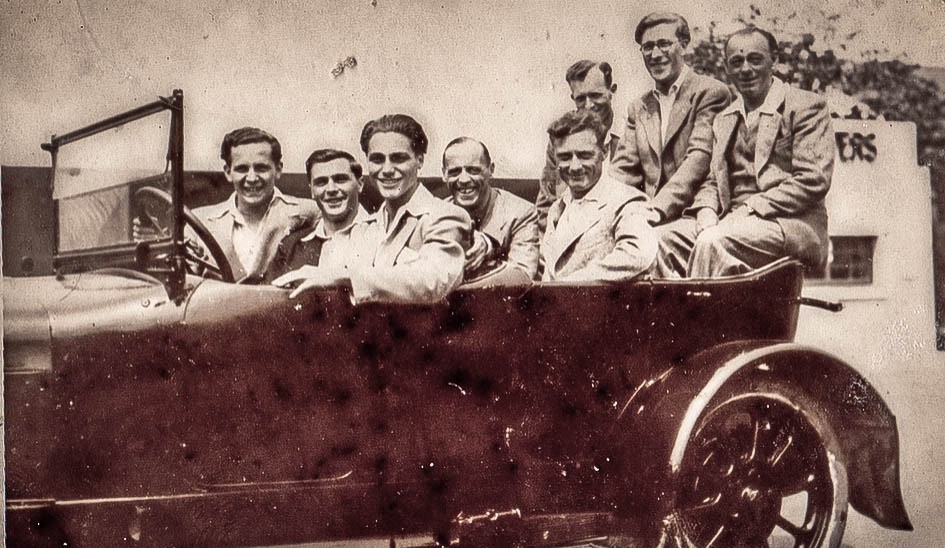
[652,24,835,280]
[270,114,474,307]
[441,137,540,285]
[608,11,733,227]
[133,126,322,283]
[259,150,372,285]
[536,59,626,237]
[539,110,660,282]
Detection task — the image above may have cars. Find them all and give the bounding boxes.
[3,90,914,548]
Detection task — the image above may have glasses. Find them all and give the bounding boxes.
[640,40,685,56]
